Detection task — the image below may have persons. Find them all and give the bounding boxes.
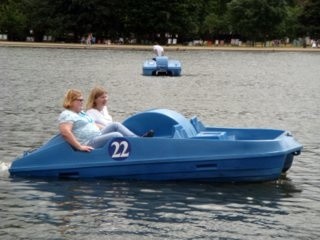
[0,29,320,52]
[152,40,165,60]
[58,88,155,153]
[84,85,114,130]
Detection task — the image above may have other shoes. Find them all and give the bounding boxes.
[142,129,155,137]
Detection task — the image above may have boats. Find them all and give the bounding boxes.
[6,108,304,185]
[142,55,182,77]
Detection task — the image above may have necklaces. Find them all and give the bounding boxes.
[77,111,93,122]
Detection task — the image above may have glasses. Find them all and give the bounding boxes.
[75,98,83,102]
